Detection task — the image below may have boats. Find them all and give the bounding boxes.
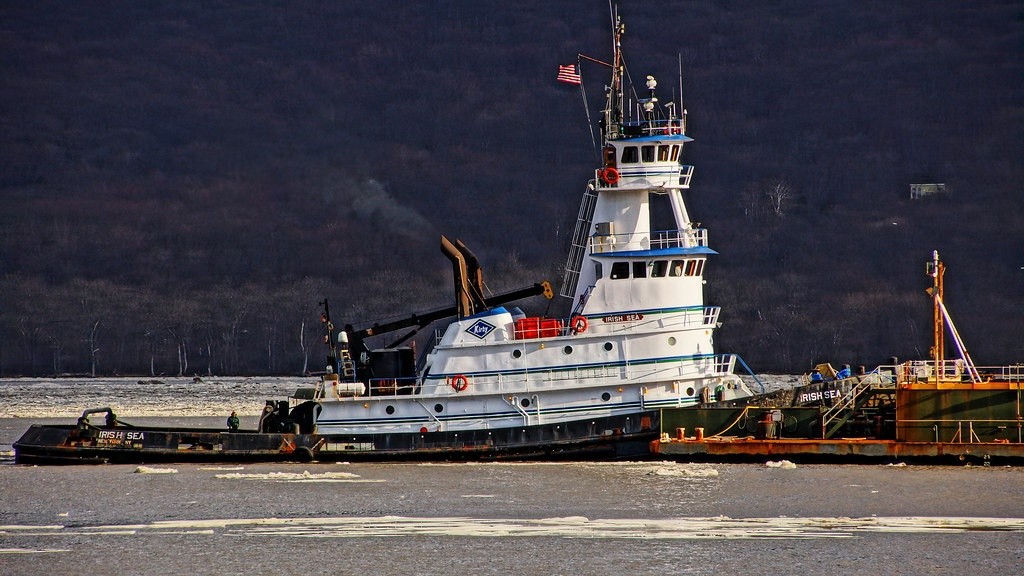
[264,0,901,463]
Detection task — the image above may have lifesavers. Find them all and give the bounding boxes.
[603,167,620,184]
[806,420,842,437]
[663,123,678,135]
[570,315,589,333]
[451,373,468,392]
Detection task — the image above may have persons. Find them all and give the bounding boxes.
[675,262,681,276]
[227,412,240,433]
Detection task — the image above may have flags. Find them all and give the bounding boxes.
[557,61,581,85]
